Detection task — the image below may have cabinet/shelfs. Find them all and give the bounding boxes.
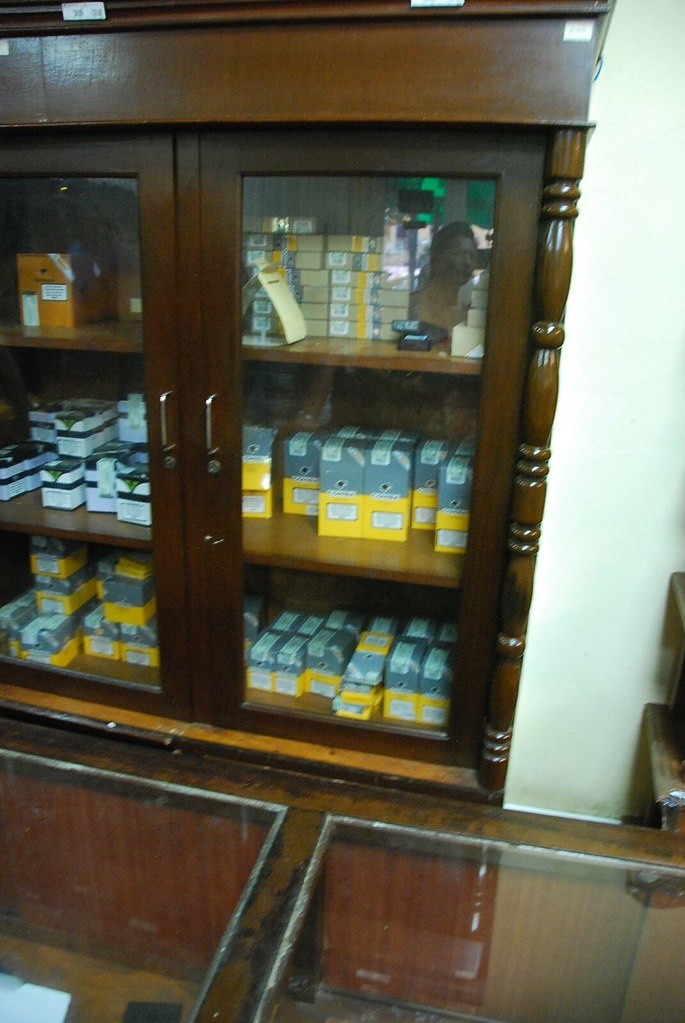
[645,572,684,833]
[0,0,615,810]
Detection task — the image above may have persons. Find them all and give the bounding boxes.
[410,218,478,335]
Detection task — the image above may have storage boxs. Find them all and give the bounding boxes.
[1,391,152,526]
[16,252,142,328]
[240,215,433,341]
[244,420,476,554]
[245,595,456,726]
[0,533,160,669]
[451,270,490,358]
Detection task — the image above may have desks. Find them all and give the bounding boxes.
[1,717,685,1023]
